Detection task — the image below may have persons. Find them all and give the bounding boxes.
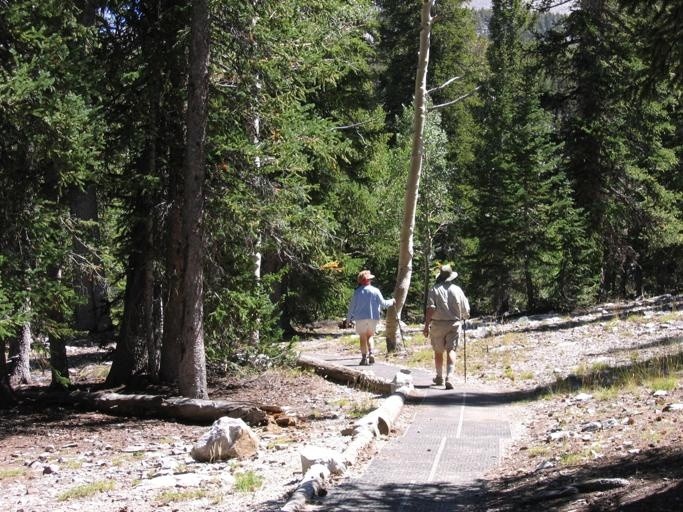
[422,264,470,391]
[346,270,396,366]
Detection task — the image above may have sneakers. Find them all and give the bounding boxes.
[358,352,375,366]
[431,375,454,390]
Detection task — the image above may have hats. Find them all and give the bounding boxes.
[357,269,375,283]
[435,264,459,283]
